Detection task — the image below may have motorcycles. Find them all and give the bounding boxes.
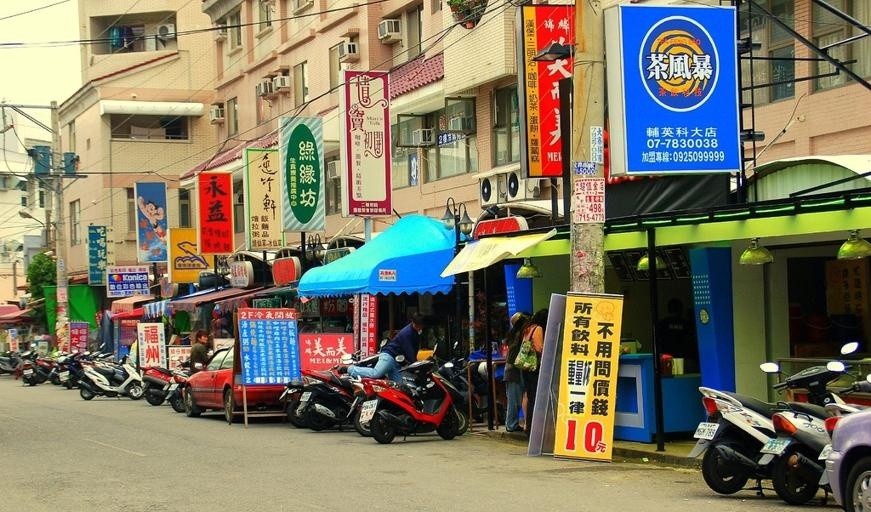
[278,337,485,446]
[687,340,870,508]
[0,343,192,414]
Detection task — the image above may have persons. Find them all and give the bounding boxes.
[337,311,427,389]
[471,290,549,438]
[659,297,689,360]
[189,318,230,372]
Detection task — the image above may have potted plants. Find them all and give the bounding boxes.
[447,0,474,29]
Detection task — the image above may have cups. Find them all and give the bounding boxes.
[672,358,684,374]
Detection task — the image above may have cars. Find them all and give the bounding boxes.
[184,345,297,418]
[824,407,871,511]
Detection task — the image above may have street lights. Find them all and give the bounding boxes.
[442,196,474,363]
[305,234,326,335]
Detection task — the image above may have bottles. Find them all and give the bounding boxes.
[660,353,673,375]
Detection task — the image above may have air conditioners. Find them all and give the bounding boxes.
[156,24,175,39]
[257,74,289,98]
[506,170,541,202]
[327,160,341,178]
[209,108,225,124]
[413,128,435,146]
[479,174,506,207]
[216,24,227,35]
[377,19,402,39]
[338,42,358,59]
[450,116,471,131]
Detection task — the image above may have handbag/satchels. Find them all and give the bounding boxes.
[512,341,538,372]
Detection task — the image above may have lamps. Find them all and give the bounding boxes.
[740,238,774,266]
[516,258,541,279]
[837,229,871,260]
[636,249,669,271]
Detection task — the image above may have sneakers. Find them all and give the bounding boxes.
[506,424,525,432]
[338,365,348,374]
